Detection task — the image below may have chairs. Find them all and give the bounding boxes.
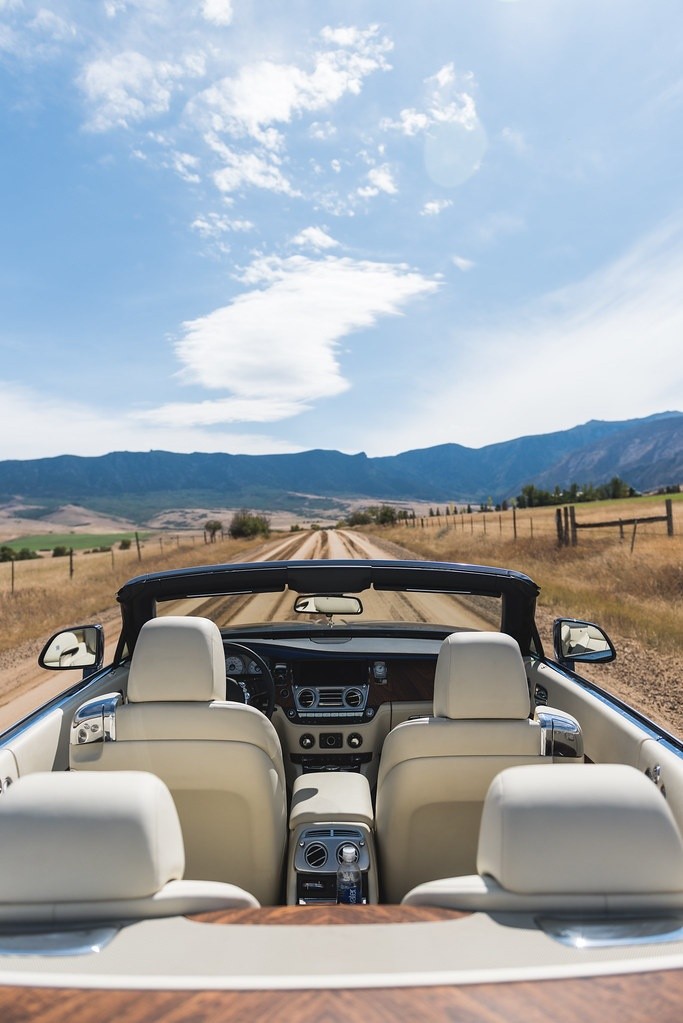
[400,763,683,918]
[0,769,259,930]
[68,616,285,915]
[370,632,584,917]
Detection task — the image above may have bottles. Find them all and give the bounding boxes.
[336,847,362,905]
